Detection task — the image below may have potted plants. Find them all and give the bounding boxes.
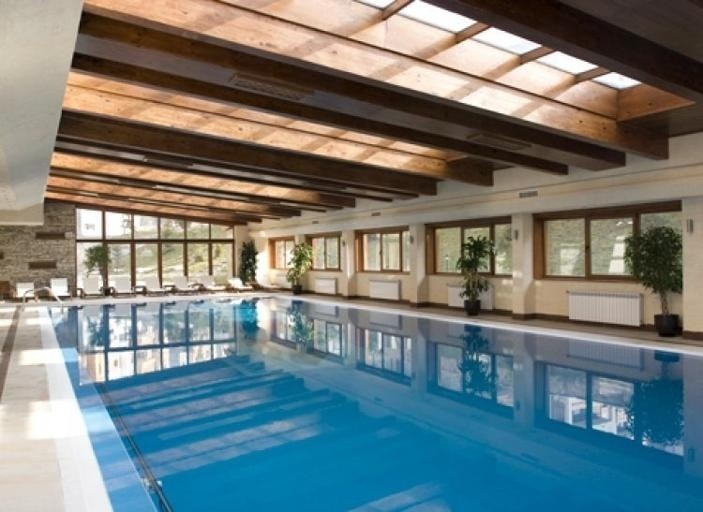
[238,240,258,291]
[284,242,313,295]
[85,244,112,295]
[239,300,259,337]
[88,306,111,346]
[624,231,682,337]
[456,326,499,398]
[286,300,313,346]
[619,350,683,449]
[457,238,497,316]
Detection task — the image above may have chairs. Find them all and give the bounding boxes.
[12,279,253,300]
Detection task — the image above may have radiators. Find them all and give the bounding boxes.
[565,290,643,327]
[445,283,493,311]
[368,278,401,302]
[315,277,337,295]
